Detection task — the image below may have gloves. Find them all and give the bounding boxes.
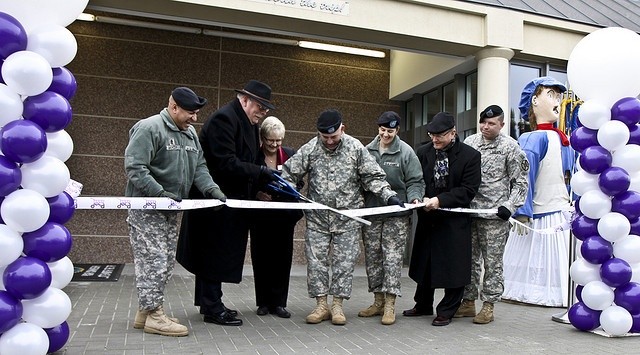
[260,165,282,181]
[159,191,182,202]
[206,187,227,202]
[387,193,405,207]
[496,205,511,221]
[270,180,283,191]
[255,190,272,201]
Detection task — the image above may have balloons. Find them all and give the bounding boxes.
[566,27,640,335]
[1,0,96,355]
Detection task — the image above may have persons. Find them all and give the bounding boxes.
[403,112,481,325]
[249,116,305,317]
[279,108,408,324]
[359,111,426,324]
[453,105,530,323]
[501,76,577,309]
[175,79,283,326]
[124,86,228,336]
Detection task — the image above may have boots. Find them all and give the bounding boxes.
[453,298,476,317]
[144,306,189,337]
[381,294,396,324]
[305,297,330,323]
[358,293,385,317]
[133,309,180,329]
[473,301,494,324]
[330,297,347,324]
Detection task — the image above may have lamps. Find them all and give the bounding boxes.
[96,15,202,34]
[203,29,299,45]
[299,41,386,58]
[77,13,95,22]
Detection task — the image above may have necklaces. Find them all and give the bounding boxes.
[263,153,276,165]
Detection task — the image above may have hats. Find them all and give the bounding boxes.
[518,77,567,122]
[234,80,276,110]
[171,87,207,111]
[426,111,455,134]
[317,109,342,134]
[480,105,503,118]
[377,111,401,128]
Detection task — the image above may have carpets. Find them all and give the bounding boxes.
[71,263,125,282]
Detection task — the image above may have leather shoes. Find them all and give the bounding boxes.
[432,314,452,325]
[269,305,291,318]
[402,305,433,317]
[200,304,238,315]
[256,305,269,315]
[204,310,243,326]
